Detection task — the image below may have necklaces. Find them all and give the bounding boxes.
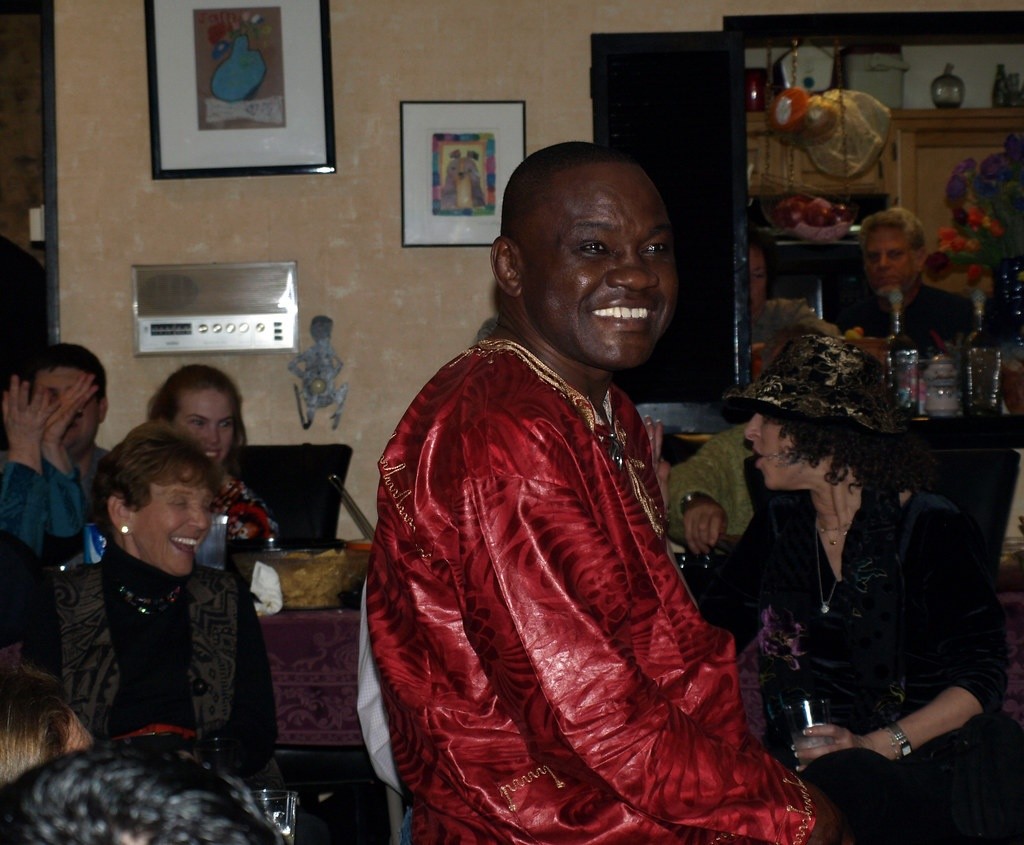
[598,419,626,471]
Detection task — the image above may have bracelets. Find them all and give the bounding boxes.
[681,491,699,502]
[879,728,901,758]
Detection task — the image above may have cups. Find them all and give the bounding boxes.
[250,790,298,845]
[782,697,831,766]
[193,738,237,778]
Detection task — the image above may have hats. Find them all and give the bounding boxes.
[722,332,907,434]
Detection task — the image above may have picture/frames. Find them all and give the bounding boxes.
[143,0,337,180]
[400,99,527,248]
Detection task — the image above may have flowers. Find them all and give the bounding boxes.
[926,133,1024,284]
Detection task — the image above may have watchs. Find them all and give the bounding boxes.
[887,720,912,757]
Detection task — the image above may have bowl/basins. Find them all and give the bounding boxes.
[759,200,861,240]
[232,547,371,609]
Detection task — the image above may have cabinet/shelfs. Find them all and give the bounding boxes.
[746,108,1024,305]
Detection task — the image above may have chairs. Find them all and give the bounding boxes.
[930,446,1019,585]
[258,607,407,845]
[630,399,731,464]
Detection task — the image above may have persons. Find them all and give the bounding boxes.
[0,658,95,787]
[640,298,846,557]
[21,423,278,778]
[147,362,286,544]
[840,207,975,346]
[674,333,1010,845]
[0,734,278,845]
[356,141,845,844]
[0,344,110,560]
[749,224,840,378]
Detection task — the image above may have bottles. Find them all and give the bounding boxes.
[993,65,1021,106]
[884,283,915,408]
[962,290,994,414]
[932,64,965,109]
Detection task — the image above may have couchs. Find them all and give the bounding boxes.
[737,591,1024,747]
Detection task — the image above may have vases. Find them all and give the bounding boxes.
[991,257,1024,345]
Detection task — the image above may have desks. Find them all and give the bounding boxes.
[223,542,372,608]
